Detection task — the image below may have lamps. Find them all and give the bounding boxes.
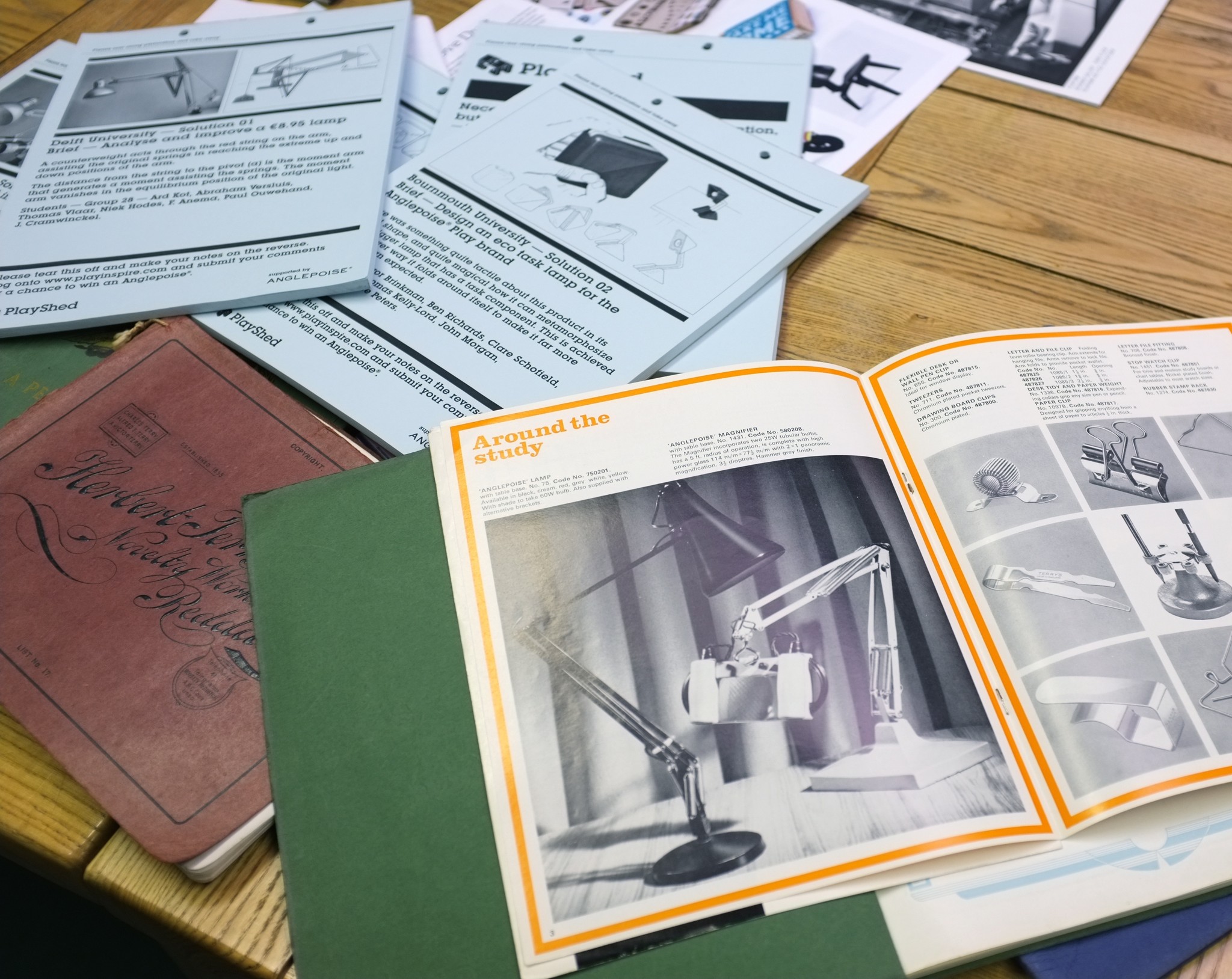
[514,480,999,886]
[82,56,218,112]
[0,98,45,125]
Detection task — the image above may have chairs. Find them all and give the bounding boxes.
[810,52,899,109]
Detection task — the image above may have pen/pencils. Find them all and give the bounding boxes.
[1122,513,1166,584]
[1175,506,1221,587]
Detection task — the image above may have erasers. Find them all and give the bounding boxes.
[724,1,813,41]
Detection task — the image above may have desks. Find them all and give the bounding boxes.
[0,0,1232,979]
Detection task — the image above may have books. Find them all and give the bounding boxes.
[2,0,1231,979]
